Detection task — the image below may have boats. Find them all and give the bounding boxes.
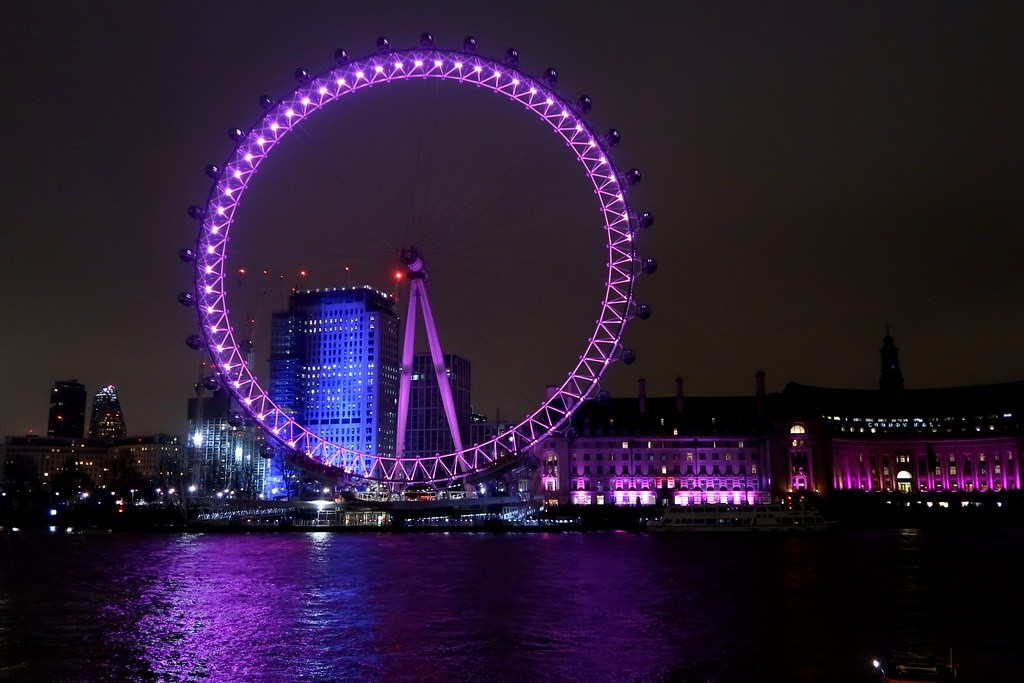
[642,505,840,534]
